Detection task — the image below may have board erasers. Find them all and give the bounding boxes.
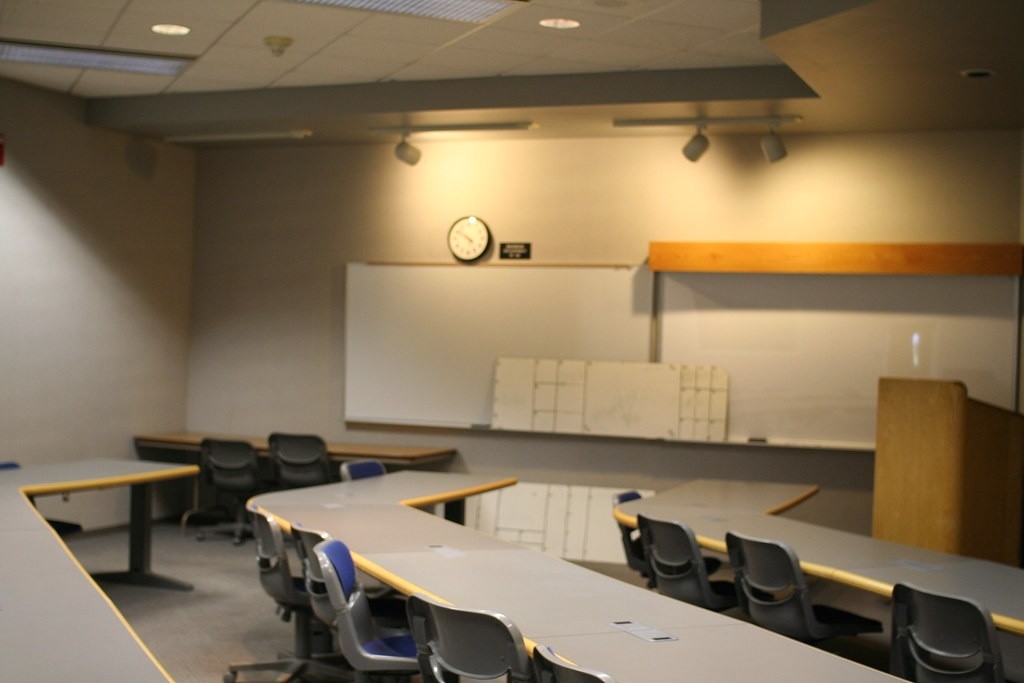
[471,424,491,430]
[747,438,767,444]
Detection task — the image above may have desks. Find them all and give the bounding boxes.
[244,469,914,682]
[134,431,457,522]
[613,478,1024,682]
[0,459,198,682]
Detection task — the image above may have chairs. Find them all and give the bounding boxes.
[724,529,883,641]
[268,431,334,491]
[0,461,82,541]
[194,437,263,545]
[221,502,418,682]
[340,458,387,482]
[636,510,736,611]
[613,489,722,589]
[406,592,534,682]
[533,643,615,682]
[890,580,1005,683]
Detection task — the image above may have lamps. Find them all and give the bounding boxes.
[682,124,709,162]
[760,125,788,163]
[394,132,421,165]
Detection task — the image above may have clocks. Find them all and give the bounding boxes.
[446,216,490,261]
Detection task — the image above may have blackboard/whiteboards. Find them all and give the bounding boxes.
[343,259,653,441]
[656,268,1015,449]
